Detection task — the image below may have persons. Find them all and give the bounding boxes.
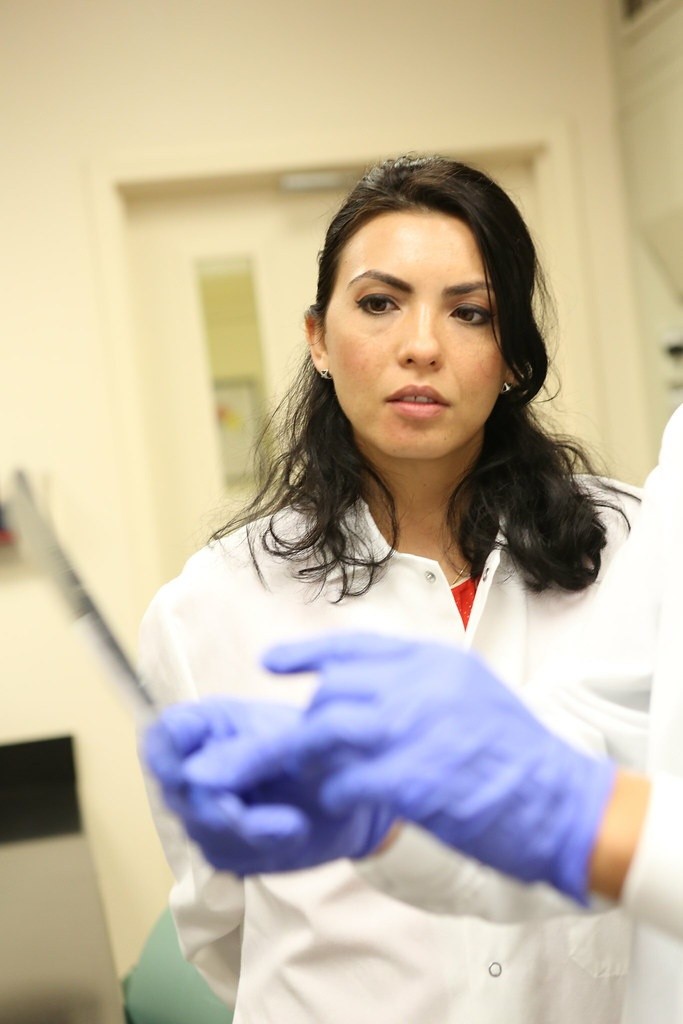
[130,155,683,1024]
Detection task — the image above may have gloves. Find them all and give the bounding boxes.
[259,631,616,910]
[138,692,399,876]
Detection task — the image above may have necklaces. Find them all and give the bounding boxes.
[447,558,471,586]
